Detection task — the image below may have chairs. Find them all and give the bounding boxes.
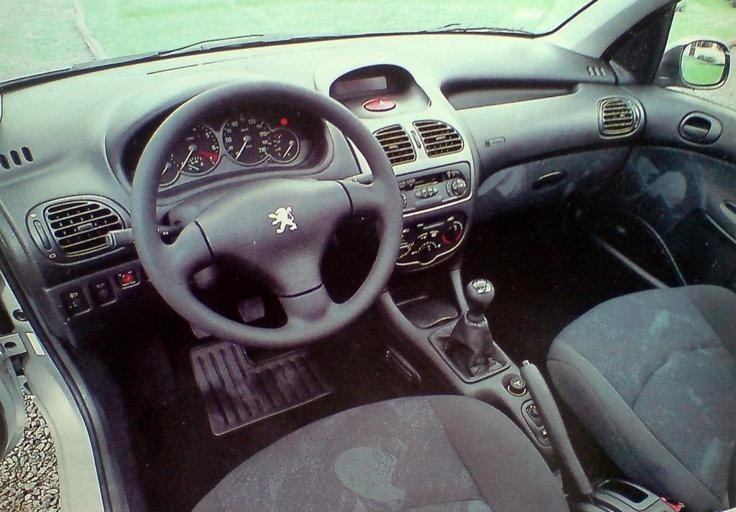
[193,393,570,512]
[546,284,735,512]
